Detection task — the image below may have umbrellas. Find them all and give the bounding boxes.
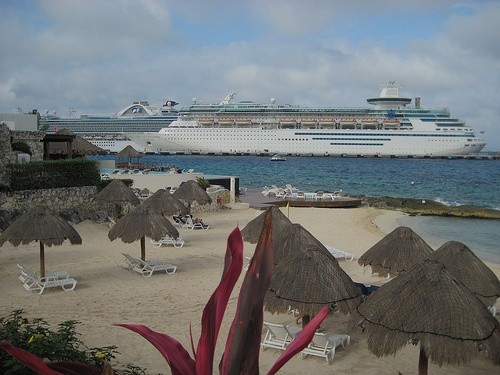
[116,145,142,158]
[0,203,82,281]
[171,180,213,214]
[426,241,500,308]
[250,223,364,329]
[96,179,140,221]
[358,226,436,278]
[143,188,190,216]
[107,203,179,269]
[347,259,500,375]
[240,206,294,243]
[48,128,110,155]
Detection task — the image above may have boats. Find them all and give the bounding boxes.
[219,119,233,125]
[384,120,399,127]
[361,119,379,128]
[320,120,335,126]
[198,118,214,125]
[234,119,251,127]
[340,121,357,127]
[280,120,294,127]
[82,134,125,140]
[300,120,317,127]
[272,154,286,161]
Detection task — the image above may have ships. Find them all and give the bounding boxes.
[121,81,488,157]
[39,99,179,155]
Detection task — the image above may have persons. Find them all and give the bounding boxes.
[178,214,205,226]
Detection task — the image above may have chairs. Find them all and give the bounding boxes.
[110,169,151,174]
[119,252,177,278]
[325,245,357,261]
[172,214,211,231]
[300,332,350,365]
[108,216,116,228]
[17,263,78,295]
[261,184,342,201]
[169,169,195,174]
[149,235,185,249]
[260,322,300,351]
